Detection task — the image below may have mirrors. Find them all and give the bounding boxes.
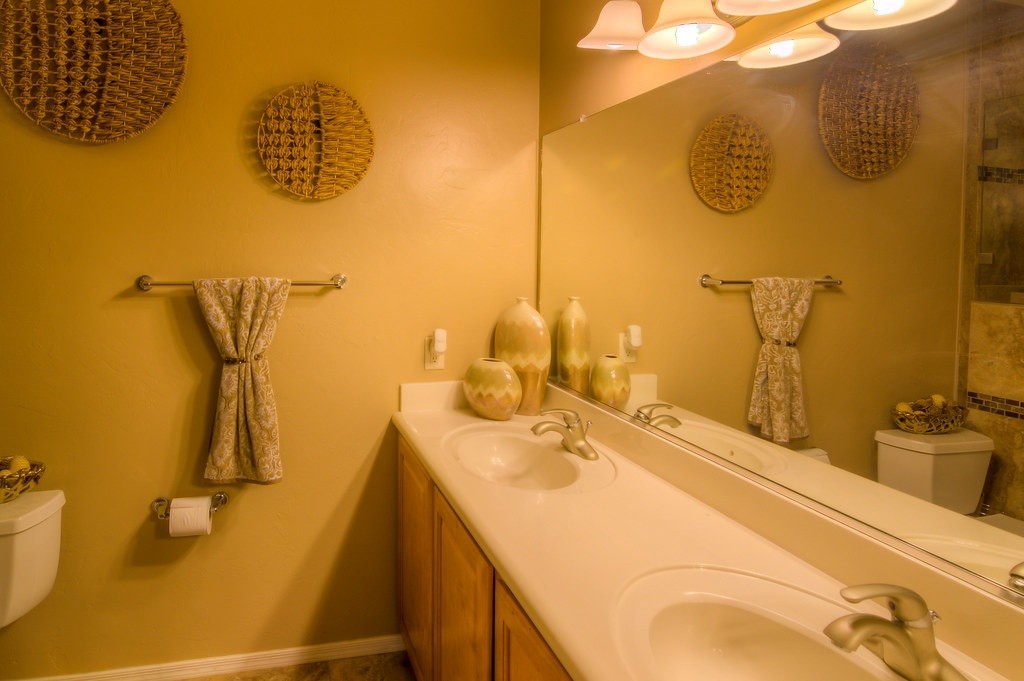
[538,0,1024,608]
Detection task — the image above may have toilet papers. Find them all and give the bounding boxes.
[169,496,213,537]
[796,448,830,465]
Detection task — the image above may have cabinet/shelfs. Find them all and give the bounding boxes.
[397,432,573,681]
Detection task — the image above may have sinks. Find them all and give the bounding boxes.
[656,418,788,482]
[611,564,907,681]
[900,535,1024,593]
[442,421,617,492]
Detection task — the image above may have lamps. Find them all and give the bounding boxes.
[738,22,840,70]
[637,0,736,60]
[823,0,958,31]
[715,0,821,17]
[576,0,646,50]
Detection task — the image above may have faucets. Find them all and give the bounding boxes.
[530,408,599,460]
[634,404,682,428]
[822,583,969,681]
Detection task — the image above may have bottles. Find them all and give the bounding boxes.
[556,293,591,398]
[494,296,552,417]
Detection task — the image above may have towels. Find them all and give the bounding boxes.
[747,277,814,443]
[192,276,292,484]
